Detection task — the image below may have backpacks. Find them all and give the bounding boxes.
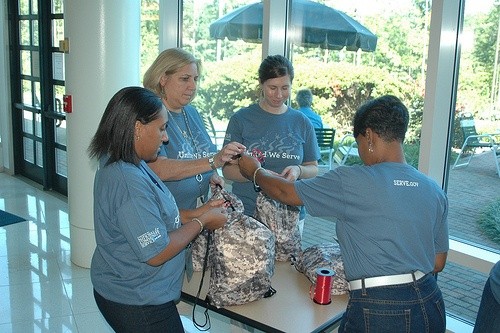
[254,190,303,262]
[193,200,275,332]
[191,183,245,272]
[291,243,349,296]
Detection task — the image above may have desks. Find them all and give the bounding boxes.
[179,261,348,333]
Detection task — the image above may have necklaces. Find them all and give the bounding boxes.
[166,107,203,183]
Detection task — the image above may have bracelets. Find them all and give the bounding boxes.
[253,167,265,188]
[191,217,203,233]
[296,164,302,180]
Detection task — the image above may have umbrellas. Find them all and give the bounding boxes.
[208,0,377,63]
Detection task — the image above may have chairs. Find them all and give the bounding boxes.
[313,129,361,169]
[451,113,500,177]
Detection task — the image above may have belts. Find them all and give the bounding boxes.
[348,270,425,291]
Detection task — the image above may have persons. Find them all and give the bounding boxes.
[87,86,228,333]
[221,55,322,239]
[142,47,246,211]
[295,89,323,148]
[237,94,450,333]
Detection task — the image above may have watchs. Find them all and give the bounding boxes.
[208,154,216,170]
